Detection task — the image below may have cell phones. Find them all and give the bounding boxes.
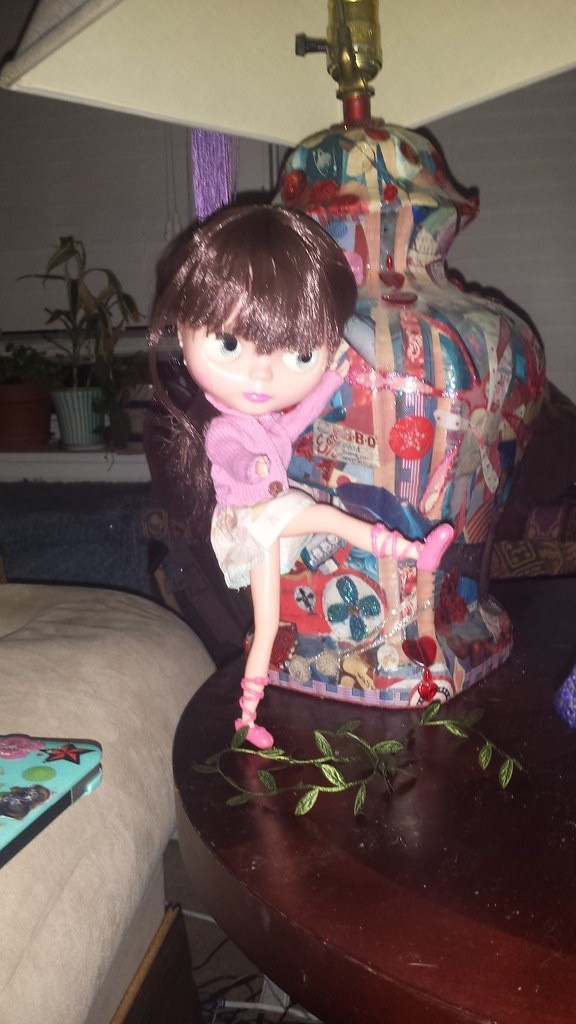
[0,734,103,870]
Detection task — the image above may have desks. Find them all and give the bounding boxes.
[171,576,576,1024]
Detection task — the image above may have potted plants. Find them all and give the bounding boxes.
[16,235,145,451]
[0,344,51,455]
[91,350,153,473]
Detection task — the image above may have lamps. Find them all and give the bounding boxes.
[0,1,575,712]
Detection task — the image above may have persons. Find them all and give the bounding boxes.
[146,205,452,751]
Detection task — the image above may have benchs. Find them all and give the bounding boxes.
[0,474,173,601]
[0,582,222,1024]
